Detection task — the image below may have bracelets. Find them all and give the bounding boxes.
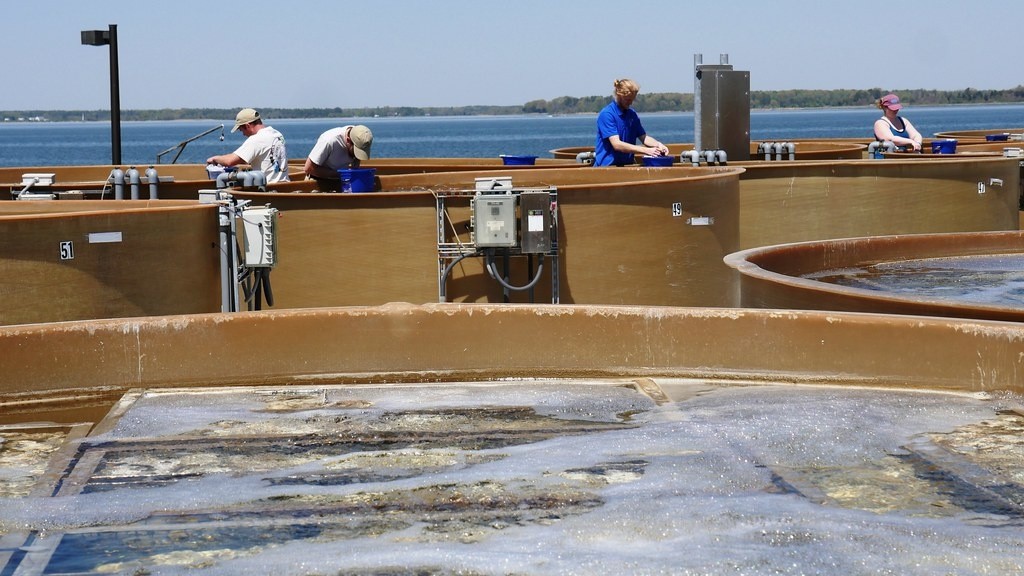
[917,141,922,145]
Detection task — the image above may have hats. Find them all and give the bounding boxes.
[350,125,373,160]
[230,108,261,134]
[881,94,903,111]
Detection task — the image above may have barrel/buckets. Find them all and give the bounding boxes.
[642,156,674,167]
[338,167,376,192]
[499,154,539,165]
[986,135,1008,141]
[932,140,957,153]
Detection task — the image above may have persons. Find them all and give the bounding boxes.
[873,94,924,151]
[304,124,374,181]
[595,79,669,167]
[207,108,291,184]
